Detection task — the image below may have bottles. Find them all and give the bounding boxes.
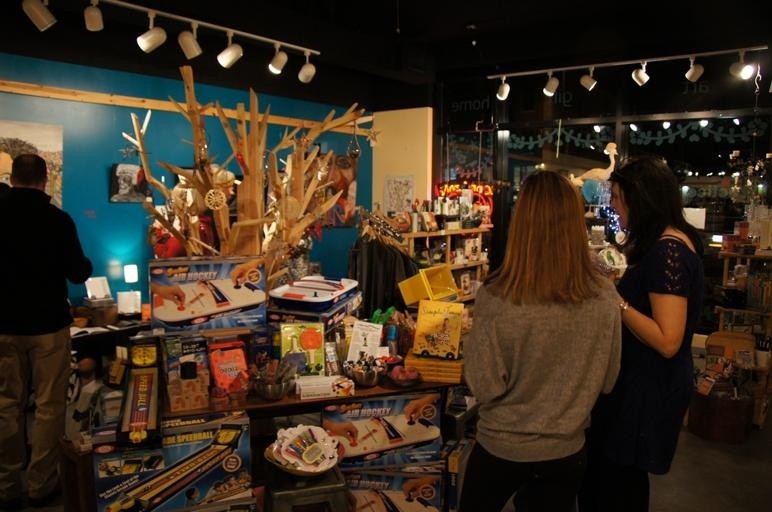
[734,222,749,240]
[722,234,741,251]
[748,235,761,251]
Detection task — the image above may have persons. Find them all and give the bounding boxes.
[230,259,265,286]
[0,155,94,506]
[150,281,186,307]
[403,394,441,421]
[323,418,359,442]
[609,155,712,512]
[460,170,625,511]
[402,477,440,497]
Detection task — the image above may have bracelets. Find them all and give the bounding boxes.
[619,298,631,322]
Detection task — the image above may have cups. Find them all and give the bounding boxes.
[754,346,769,367]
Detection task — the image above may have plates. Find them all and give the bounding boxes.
[263,442,347,477]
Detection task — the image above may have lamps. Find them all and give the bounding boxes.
[486,45,769,102]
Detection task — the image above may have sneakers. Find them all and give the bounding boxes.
[0,458,72,512]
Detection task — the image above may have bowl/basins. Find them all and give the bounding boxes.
[352,366,386,387]
[254,381,290,402]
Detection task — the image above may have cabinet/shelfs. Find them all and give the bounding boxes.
[714,248,772,331]
[402,226,492,303]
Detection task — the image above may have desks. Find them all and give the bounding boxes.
[63,373,469,511]
